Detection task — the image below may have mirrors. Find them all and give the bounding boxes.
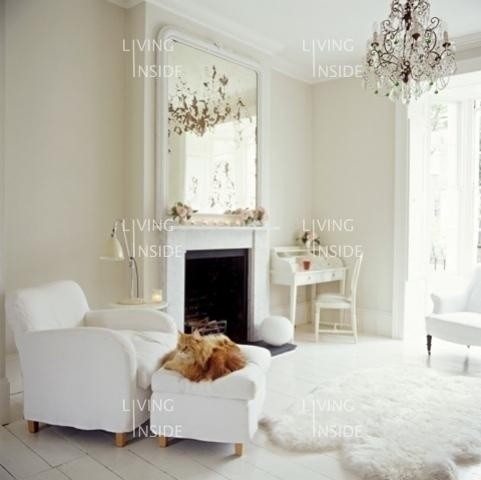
[153,24,267,228]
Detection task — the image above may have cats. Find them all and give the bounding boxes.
[162,327,247,382]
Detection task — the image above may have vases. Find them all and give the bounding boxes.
[304,242,311,256]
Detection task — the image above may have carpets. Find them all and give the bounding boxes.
[267,376,481,480]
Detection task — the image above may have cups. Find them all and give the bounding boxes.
[152,289,162,304]
[303,259,311,270]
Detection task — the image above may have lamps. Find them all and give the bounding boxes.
[365,0,458,106]
[101,218,154,302]
[167,56,255,137]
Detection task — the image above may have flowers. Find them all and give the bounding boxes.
[297,231,320,245]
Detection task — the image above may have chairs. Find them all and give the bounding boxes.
[314,251,365,344]
[424,279,481,355]
[6,275,181,447]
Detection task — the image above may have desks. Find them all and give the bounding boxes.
[270,245,347,345]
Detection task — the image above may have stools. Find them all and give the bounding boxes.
[147,343,270,457]
